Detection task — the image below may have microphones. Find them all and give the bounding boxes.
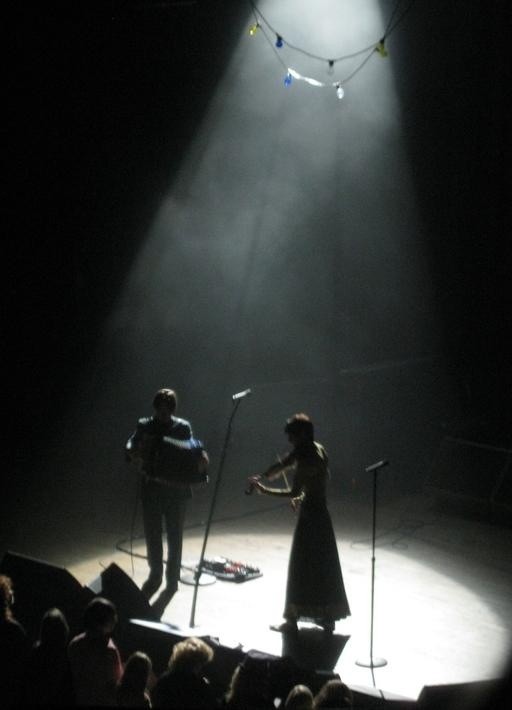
[362,460,389,474]
[232,388,252,401]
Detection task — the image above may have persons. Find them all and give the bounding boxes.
[1,575,354,710]
[123,386,210,594]
[248,412,352,640]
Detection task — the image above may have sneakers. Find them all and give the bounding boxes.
[142,571,162,592]
[166,577,177,593]
[271,623,297,632]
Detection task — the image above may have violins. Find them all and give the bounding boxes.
[246,447,296,495]
